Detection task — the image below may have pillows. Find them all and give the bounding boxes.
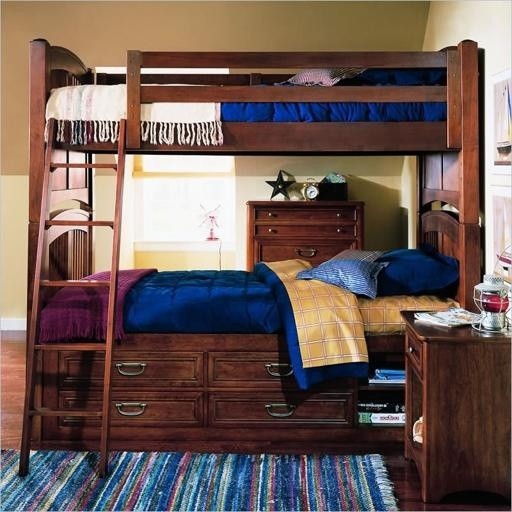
[298,245,461,297]
[283,67,368,87]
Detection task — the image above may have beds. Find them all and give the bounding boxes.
[25,38,484,455]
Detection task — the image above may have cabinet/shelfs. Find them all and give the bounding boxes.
[59,348,402,427]
[400,309,512,500]
[245,198,367,272]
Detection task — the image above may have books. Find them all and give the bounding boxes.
[413,305,481,327]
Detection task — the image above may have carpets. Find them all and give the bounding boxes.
[0,447,399,512]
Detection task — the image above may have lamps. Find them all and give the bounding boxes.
[473,273,512,334]
